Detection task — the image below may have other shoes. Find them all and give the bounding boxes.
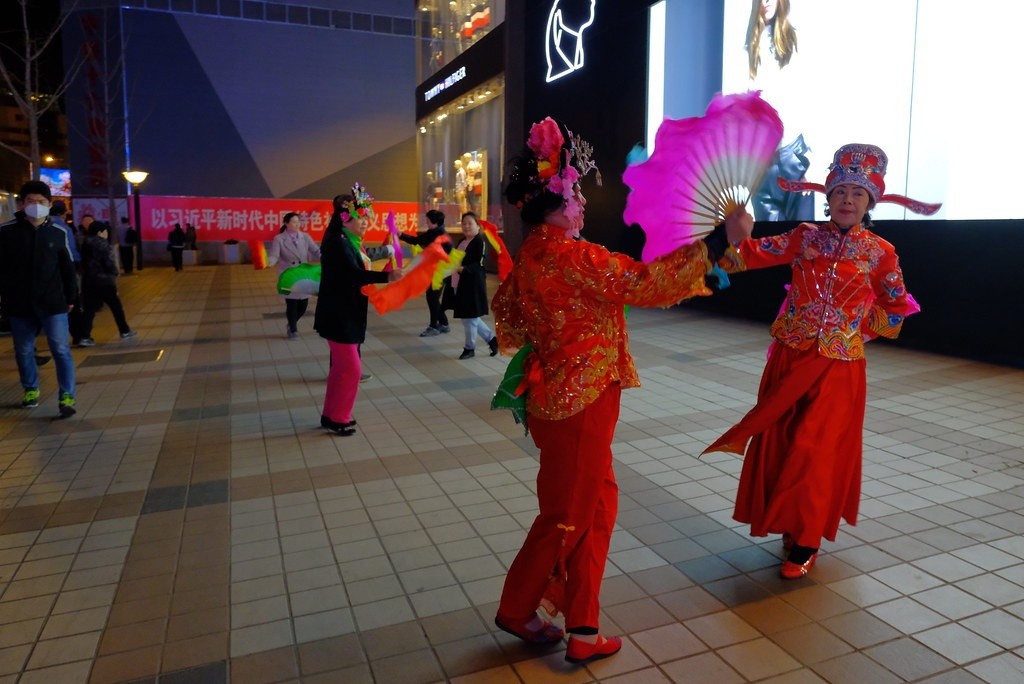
[564,634,622,664]
[359,373,372,382]
[320,416,357,436]
[288,330,299,341]
[494,617,564,644]
[121,330,137,339]
[419,327,439,337]
[33,355,52,367]
[781,551,816,577]
[439,324,450,333]
[458,348,475,359]
[489,337,499,356]
[80,338,96,346]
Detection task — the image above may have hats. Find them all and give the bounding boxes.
[778,144,940,214]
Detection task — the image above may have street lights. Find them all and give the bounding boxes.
[122,168,150,272]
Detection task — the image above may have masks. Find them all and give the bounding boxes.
[24,204,50,217]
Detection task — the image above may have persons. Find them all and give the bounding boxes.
[473,154,484,211]
[453,160,468,213]
[398,208,454,336]
[314,191,406,436]
[0,181,84,414]
[265,209,322,338]
[464,152,479,216]
[423,172,436,212]
[116,216,134,273]
[696,143,943,580]
[745,0,817,225]
[490,114,752,664]
[73,214,138,346]
[185,223,199,251]
[166,224,185,272]
[444,212,504,359]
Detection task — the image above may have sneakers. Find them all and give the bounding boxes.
[22,388,40,406]
[58,388,77,415]
